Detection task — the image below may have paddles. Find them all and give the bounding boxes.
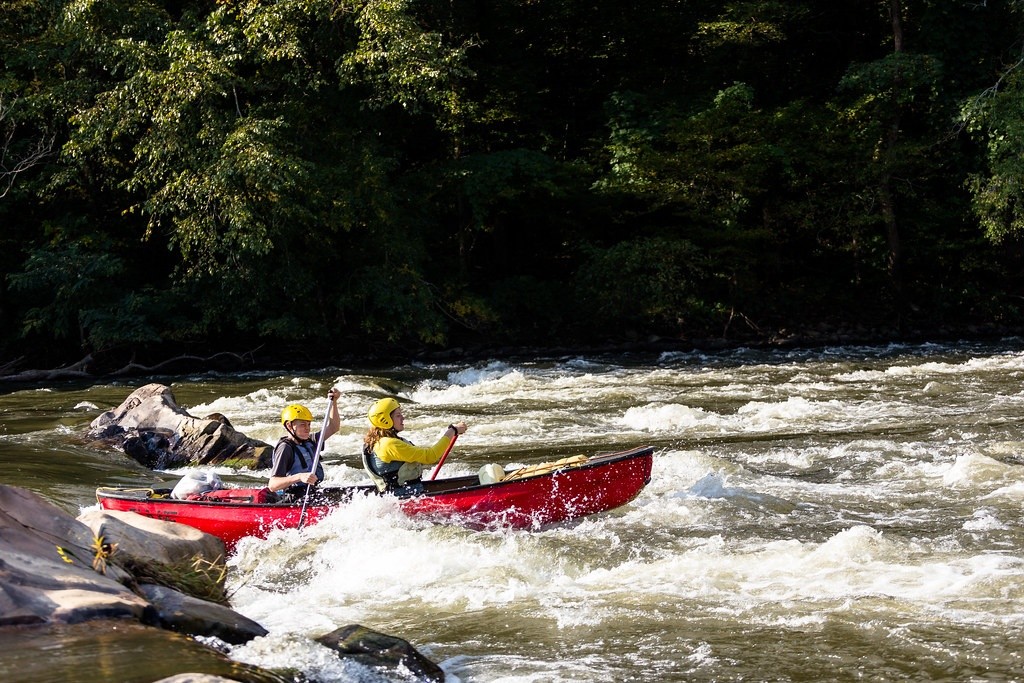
[297,390,335,529]
[430,433,460,480]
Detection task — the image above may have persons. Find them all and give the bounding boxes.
[268,387,341,504]
[362,397,468,497]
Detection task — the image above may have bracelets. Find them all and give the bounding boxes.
[448,423,458,436]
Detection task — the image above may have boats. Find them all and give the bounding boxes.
[91,441,654,559]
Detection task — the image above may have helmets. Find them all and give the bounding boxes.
[282,404,314,424]
[367,397,401,429]
